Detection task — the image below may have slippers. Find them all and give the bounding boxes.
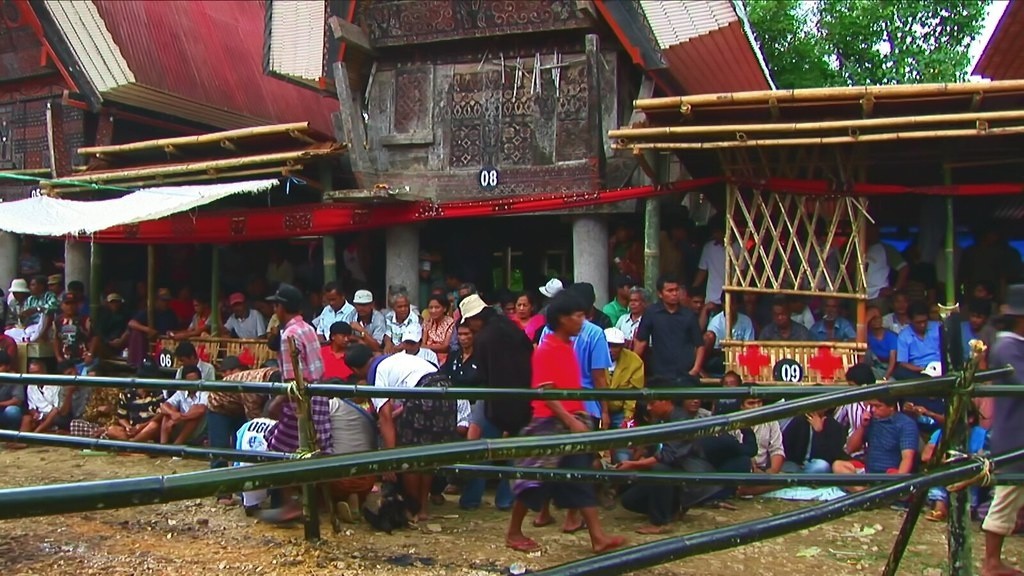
[924,509,948,522]
[215,479,739,553]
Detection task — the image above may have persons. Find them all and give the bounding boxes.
[952,219,1024,293]
[0,209,993,536]
[504,290,629,556]
[982,283,1023,576]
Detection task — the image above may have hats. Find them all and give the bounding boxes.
[1000,284,1024,316]
[919,360,942,377]
[6,271,626,358]
